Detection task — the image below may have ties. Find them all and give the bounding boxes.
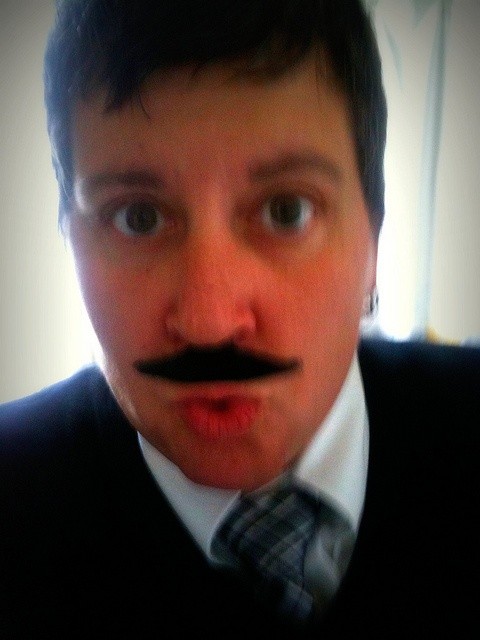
[211,484,333,638]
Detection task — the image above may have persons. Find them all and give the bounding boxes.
[1,1,480,639]
